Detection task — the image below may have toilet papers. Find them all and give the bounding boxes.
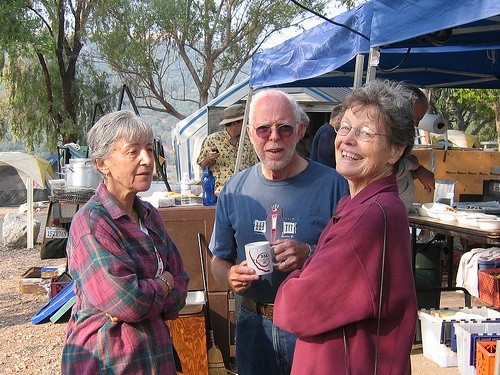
[416,113,448,135]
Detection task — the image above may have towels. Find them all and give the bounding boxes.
[456,246,499,296]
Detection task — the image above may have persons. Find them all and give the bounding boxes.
[396,85,435,213]
[273,78,419,375]
[197,104,261,206]
[311,104,346,169]
[61,109,190,375]
[209,86,351,375]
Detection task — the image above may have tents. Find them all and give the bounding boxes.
[0,152,56,249]
[233,0,500,177]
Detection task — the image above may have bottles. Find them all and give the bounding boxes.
[201,166,216,206]
[180,172,191,207]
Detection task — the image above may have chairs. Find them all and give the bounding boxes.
[413,178,457,287]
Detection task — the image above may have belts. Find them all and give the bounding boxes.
[239,295,274,323]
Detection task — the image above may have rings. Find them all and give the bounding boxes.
[284,261,287,268]
[243,282,248,287]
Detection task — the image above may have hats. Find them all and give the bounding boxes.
[218,104,246,125]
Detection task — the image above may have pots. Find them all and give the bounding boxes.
[58,157,104,193]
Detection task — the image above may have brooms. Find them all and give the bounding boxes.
[196,231,227,375]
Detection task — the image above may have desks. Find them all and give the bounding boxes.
[47,196,89,273]
[408,206,500,314]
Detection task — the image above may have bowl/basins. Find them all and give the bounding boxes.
[47,179,66,188]
[411,201,500,230]
[140,196,176,209]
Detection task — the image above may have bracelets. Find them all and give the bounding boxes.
[158,275,172,293]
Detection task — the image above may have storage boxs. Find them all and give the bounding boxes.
[49,274,75,300]
[419,252,500,375]
[20,266,64,294]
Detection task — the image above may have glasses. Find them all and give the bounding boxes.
[251,122,294,139]
[334,121,388,142]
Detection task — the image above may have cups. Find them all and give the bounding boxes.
[244,241,282,275]
